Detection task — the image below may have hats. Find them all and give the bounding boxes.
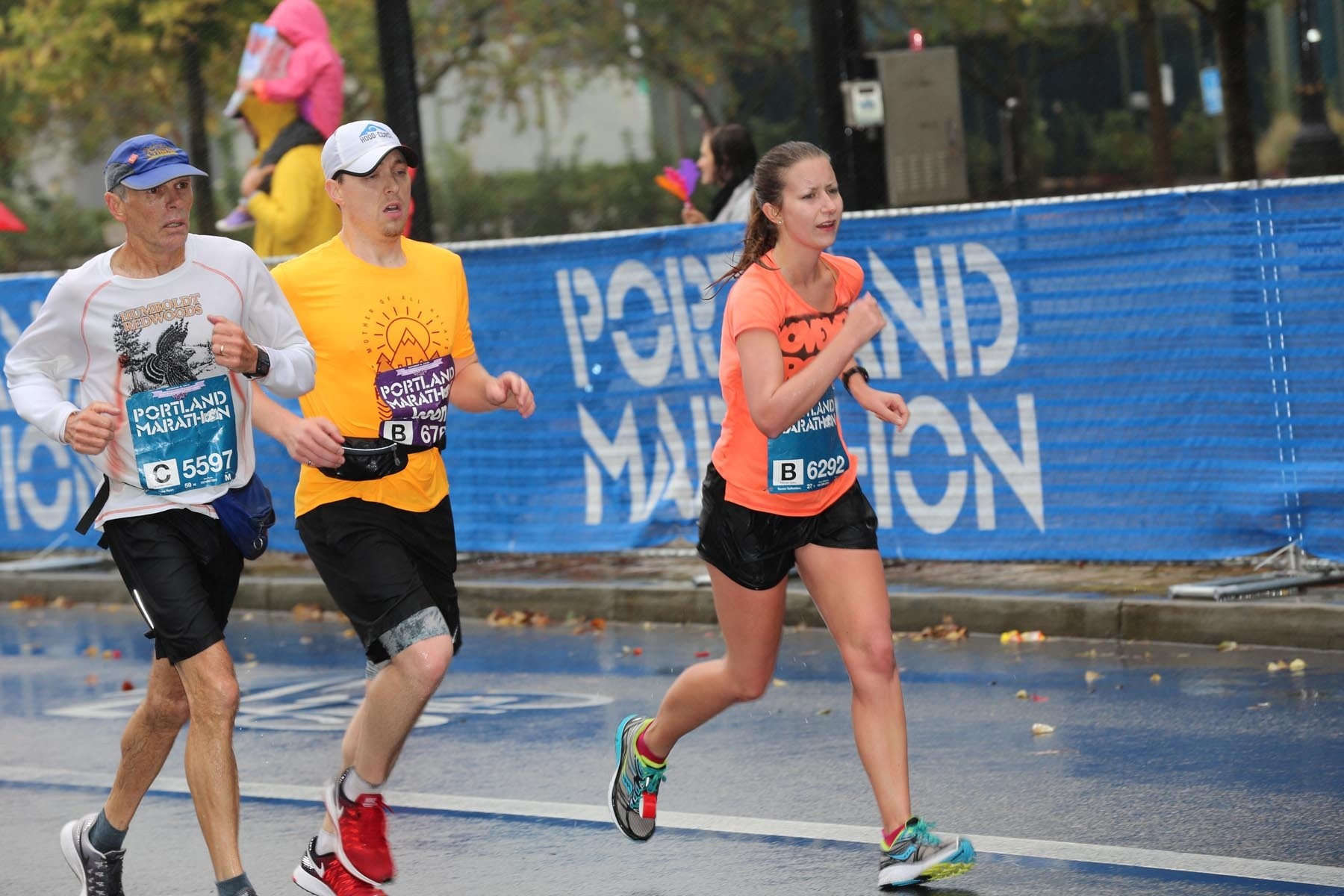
[104,134,209,192]
[321,119,421,182]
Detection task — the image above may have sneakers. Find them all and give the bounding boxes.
[292,836,389,896]
[608,714,668,844]
[323,765,397,885]
[877,818,976,889]
[61,813,127,896]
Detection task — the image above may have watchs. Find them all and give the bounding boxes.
[242,347,270,379]
[841,367,869,392]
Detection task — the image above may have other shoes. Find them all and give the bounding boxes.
[215,210,256,232]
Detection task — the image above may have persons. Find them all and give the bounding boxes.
[249,118,536,896]
[3,137,316,895]
[216,0,342,255]
[1256,82,1344,179]
[680,124,760,223]
[609,142,975,889]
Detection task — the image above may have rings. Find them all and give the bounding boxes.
[219,345,224,356]
[307,460,311,466]
